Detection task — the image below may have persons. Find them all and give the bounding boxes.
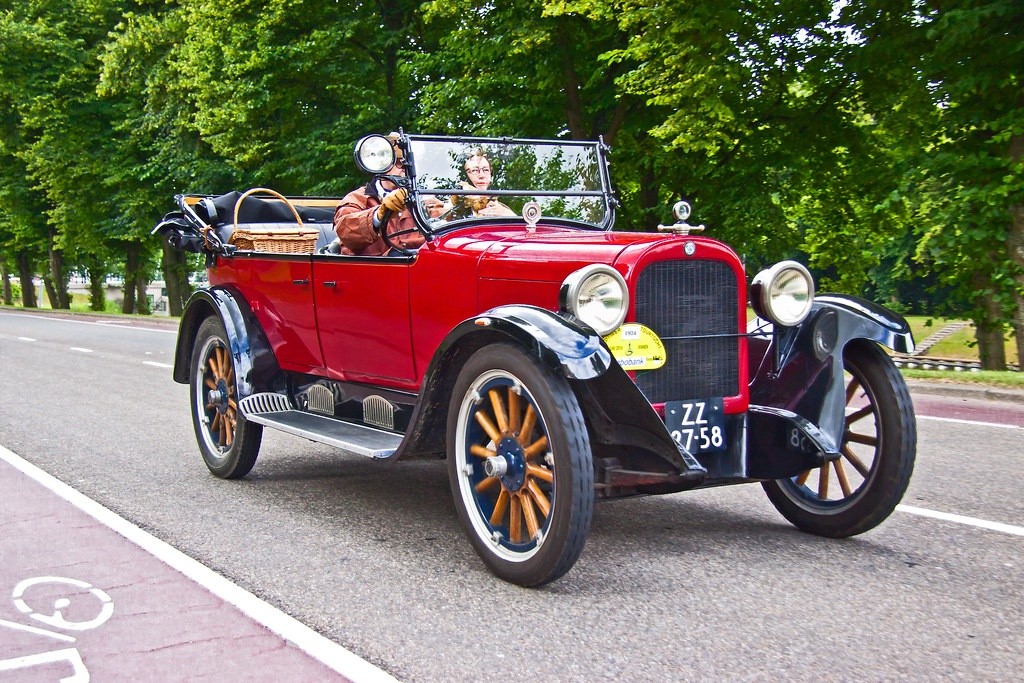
[444,149,517,216]
[332,132,479,257]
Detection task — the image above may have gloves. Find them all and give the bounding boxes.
[378,188,409,221]
[451,181,489,212]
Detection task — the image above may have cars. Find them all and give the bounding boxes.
[151,124,917,587]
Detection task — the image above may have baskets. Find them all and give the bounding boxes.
[227,187,320,254]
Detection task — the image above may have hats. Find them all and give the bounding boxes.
[383,132,426,161]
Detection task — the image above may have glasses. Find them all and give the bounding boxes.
[464,166,493,176]
[395,158,406,170]
